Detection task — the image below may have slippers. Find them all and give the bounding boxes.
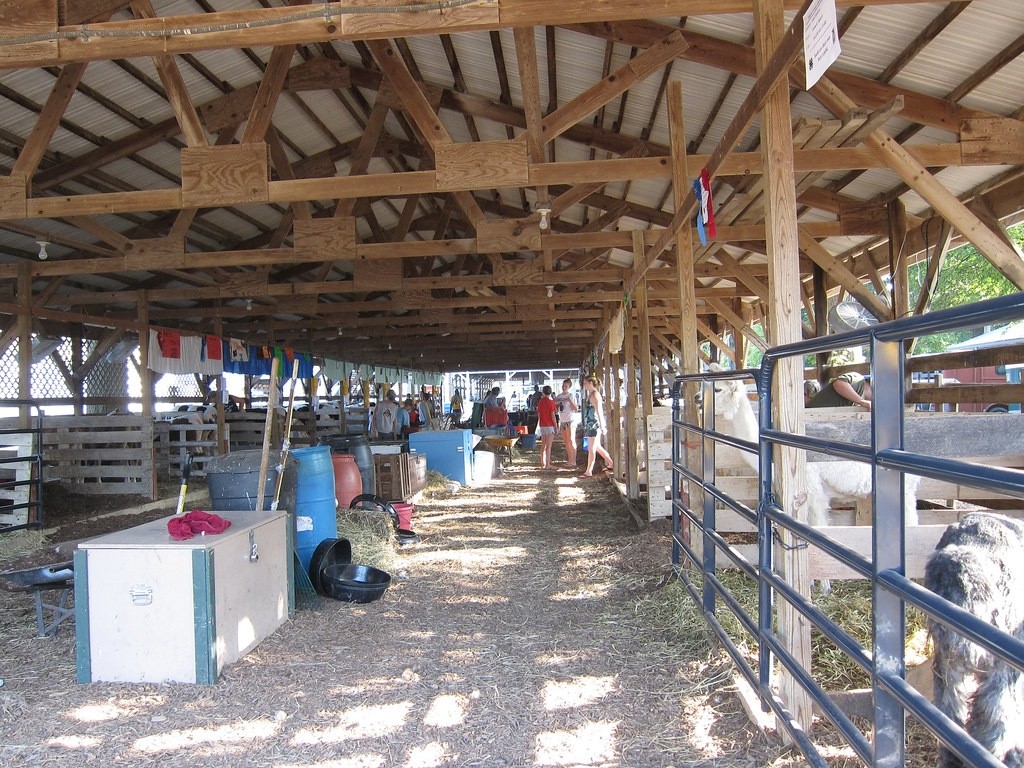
[602,467,612,472]
[561,464,579,468]
[579,474,592,478]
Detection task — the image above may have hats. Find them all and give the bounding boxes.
[405,399,414,405]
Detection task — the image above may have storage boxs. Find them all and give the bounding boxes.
[409,428,473,485]
[521,434,537,448]
[72,509,295,685]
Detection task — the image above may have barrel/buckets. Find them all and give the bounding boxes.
[331,454,362,509]
[392,503,415,531]
[203,448,297,552]
[290,444,337,594]
[582,437,588,452]
[520,434,537,449]
[316,432,376,495]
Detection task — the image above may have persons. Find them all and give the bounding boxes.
[578,373,613,478]
[536,386,558,468]
[512,391,517,398]
[804,380,821,402]
[805,371,871,412]
[413,393,434,426]
[396,394,420,439]
[555,378,578,467]
[482,387,509,428]
[449,389,464,423]
[533,385,542,410]
[373,390,398,441]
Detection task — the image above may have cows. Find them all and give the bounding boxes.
[64,395,372,482]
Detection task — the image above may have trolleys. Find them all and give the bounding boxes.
[0,531,115,637]
[485,435,520,463]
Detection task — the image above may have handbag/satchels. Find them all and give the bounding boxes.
[587,430,597,436]
[369,419,378,438]
[484,406,507,427]
[535,417,541,440]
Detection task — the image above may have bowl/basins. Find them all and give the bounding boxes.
[322,563,391,604]
[484,435,520,447]
[309,537,352,594]
